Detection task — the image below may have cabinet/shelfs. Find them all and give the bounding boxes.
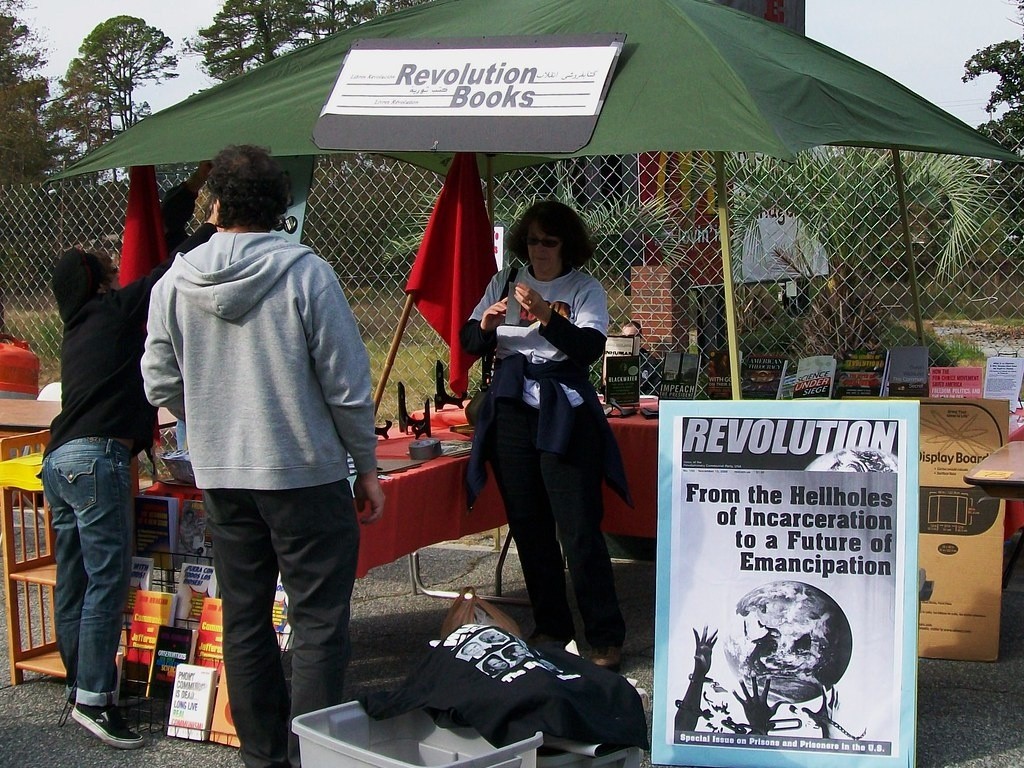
[0,428,66,684]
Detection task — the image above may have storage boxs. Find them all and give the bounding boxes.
[291,701,543,768]
[842,394,1010,662]
[537,746,644,768]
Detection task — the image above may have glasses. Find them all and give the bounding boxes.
[526,237,559,248]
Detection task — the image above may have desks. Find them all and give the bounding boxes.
[0,399,62,526]
[410,402,657,561]
[144,428,530,606]
[963,440,1024,501]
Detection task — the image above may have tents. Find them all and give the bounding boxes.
[55,0,1024,384]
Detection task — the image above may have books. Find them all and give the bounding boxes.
[119,495,296,747]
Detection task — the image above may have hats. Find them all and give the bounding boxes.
[51,249,88,321]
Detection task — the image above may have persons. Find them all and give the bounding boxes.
[675,626,842,742]
[36,159,222,749]
[140,146,387,768]
[179,510,205,552]
[458,201,626,670]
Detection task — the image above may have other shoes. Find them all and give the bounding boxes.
[590,643,620,669]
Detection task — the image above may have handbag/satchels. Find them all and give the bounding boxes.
[464,267,517,425]
[440,587,521,646]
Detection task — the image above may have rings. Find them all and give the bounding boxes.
[525,300,531,305]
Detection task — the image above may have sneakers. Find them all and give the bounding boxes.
[71,703,144,749]
